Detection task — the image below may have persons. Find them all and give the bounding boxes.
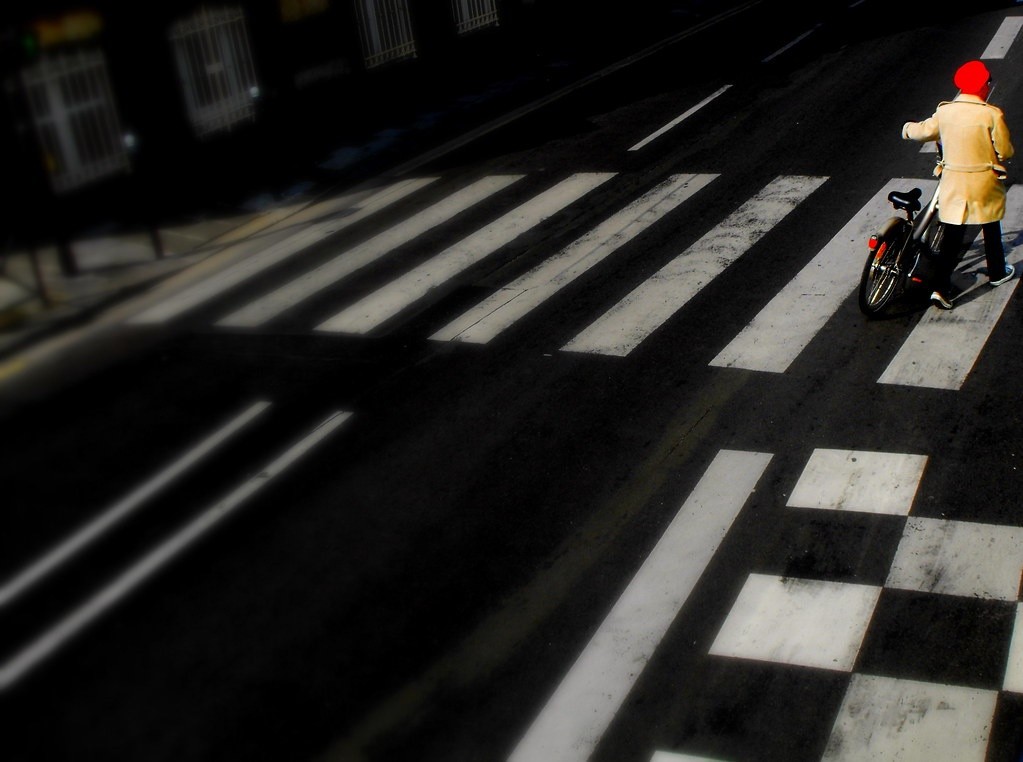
[901,61,1016,309]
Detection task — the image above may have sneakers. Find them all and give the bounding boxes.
[992,264,1015,286]
[930,291,953,309]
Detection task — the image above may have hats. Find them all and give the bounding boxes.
[953,61,991,93]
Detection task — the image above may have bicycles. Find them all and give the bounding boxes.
[858,142,1010,322]
